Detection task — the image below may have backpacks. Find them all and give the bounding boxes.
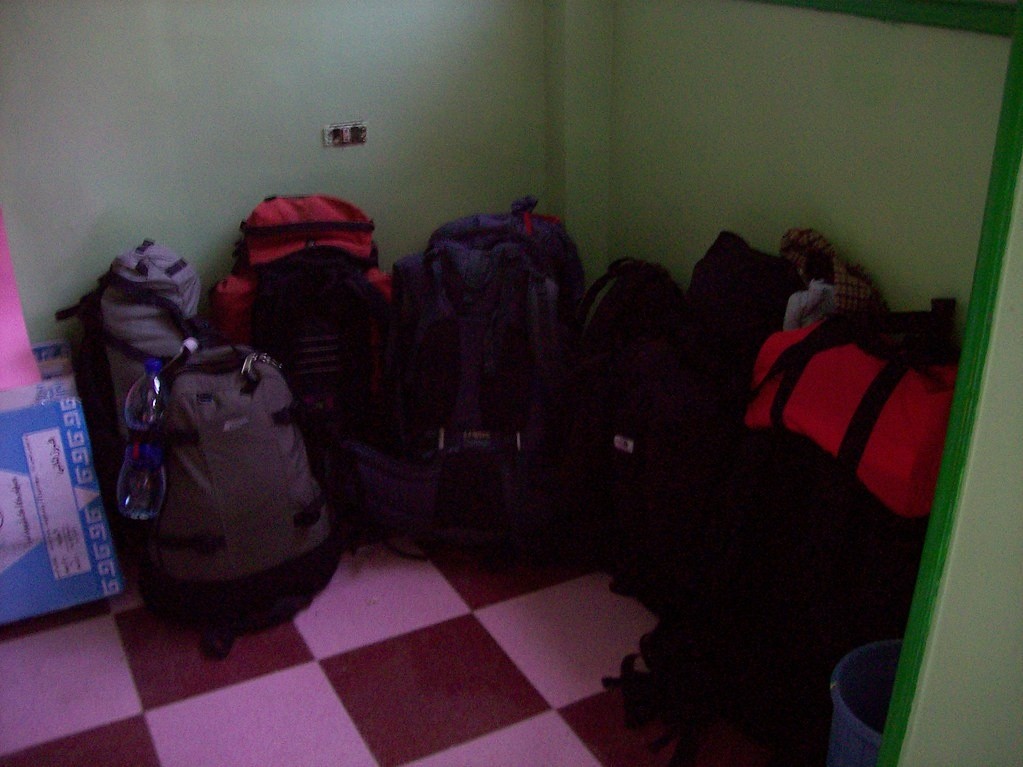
[54,195,963,767]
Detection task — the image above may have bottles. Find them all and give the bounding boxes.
[116,360,169,518]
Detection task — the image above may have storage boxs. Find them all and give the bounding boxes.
[0,340,126,624]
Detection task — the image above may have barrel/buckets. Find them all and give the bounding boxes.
[829,640,904,767]
